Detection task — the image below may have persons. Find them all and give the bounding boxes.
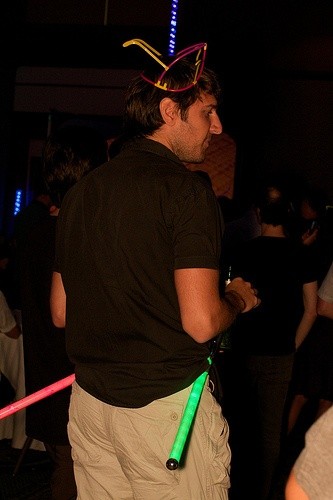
[0,56,333,500]
[49,57,262,500]
